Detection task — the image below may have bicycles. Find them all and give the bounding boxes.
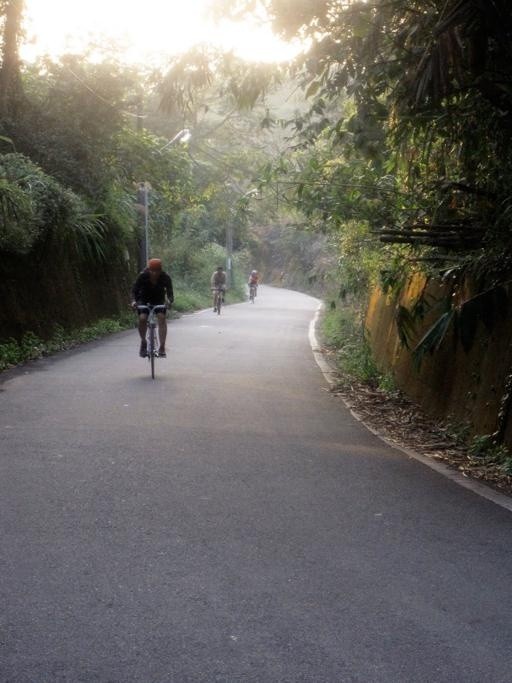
[135,303,168,379]
[212,286,225,314]
[248,283,257,303]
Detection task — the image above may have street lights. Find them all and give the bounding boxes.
[227,187,258,289]
[145,126,192,270]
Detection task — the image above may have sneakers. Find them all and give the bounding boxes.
[158,349,166,357]
[140,340,148,357]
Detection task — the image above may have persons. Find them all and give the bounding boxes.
[211,265,228,312]
[247,269,260,301]
[133,257,176,357]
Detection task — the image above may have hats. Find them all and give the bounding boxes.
[149,259,162,271]
